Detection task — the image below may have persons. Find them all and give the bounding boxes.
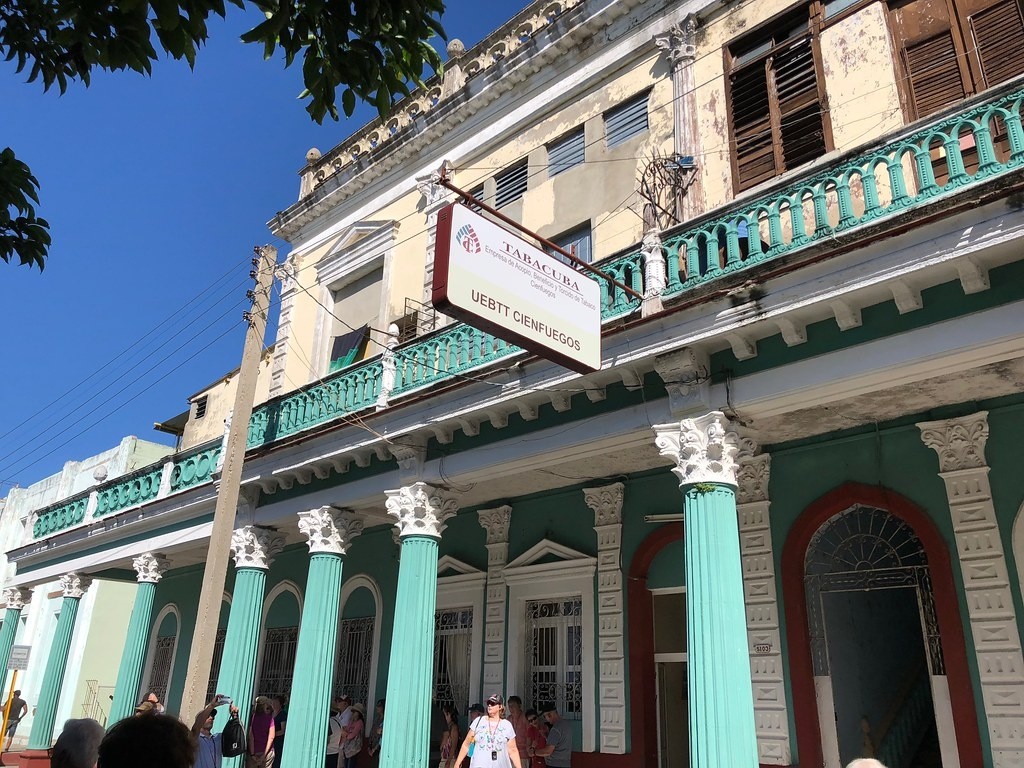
[51,691,197,768]
[326,694,386,768]
[429,702,485,768]
[507,696,531,768]
[453,694,523,768]
[529,704,572,768]
[191,694,244,768]
[244,695,287,768]
[525,710,550,768]
[0,690,27,752]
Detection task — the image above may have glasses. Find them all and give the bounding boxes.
[47,748,55,758]
[528,716,538,724]
[542,713,550,718]
[486,700,497,706]
[350,709,358,713]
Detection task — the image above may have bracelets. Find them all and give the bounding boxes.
[250,754,255,756]
[534,750,536,756]
[233,715,237,716]
[156,700,159,703]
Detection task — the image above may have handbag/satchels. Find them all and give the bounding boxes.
[222,712,244,757]
[466,715,484,757]
[344,731,362,758]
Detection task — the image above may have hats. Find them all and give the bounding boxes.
[538,703,555,716]
[467,703,484,713]
[484,693,503,704]
[195,709,218,722]
[335,695,350,702]
[255,697,271,705]
[13,690,21,695]
[348,702,363,715]
[134,702,152,711]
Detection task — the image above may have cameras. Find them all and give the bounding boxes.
[492,751,497,760]
[218,696,230,703]
